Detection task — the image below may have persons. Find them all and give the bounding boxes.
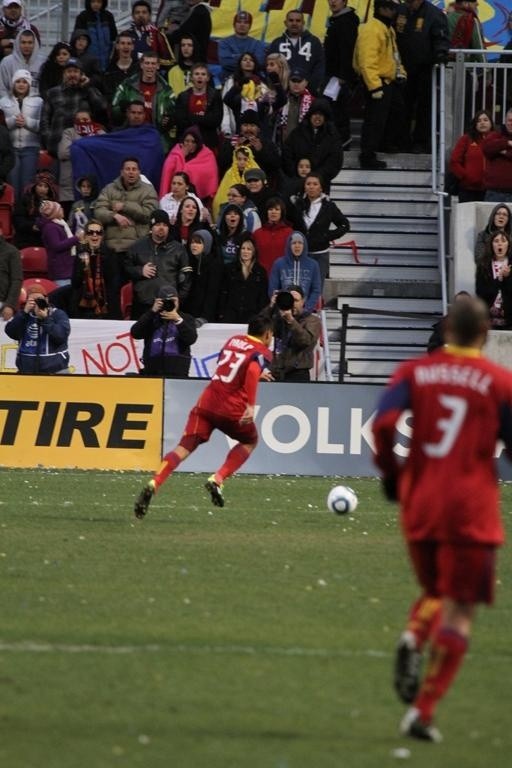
[451,108,511,329]
[1,0,351,382]
[134,312,275,519]
[353,0,485,170]
[372,298,512,744]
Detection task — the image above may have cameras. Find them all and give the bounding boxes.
[159,298,175,312]
[273,290,294,310]
[32,299,46,316]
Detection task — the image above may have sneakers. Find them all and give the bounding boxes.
[398,706,444,744]
[206,473,225,508]
[392,629,421,705]
[359,158,388,170]
[134,479,156,520]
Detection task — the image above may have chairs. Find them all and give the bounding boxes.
[20,278,59,303]
[120,282,133,319]
[18,246,49,273]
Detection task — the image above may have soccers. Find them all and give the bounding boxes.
[327,486,358,516]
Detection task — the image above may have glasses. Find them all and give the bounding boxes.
[86,229,105,236]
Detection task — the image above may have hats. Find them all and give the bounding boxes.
[26,284,48,297]
[12,68,32,86]
[158,285,179,299]
[239,109,260,126]
[3,0,22,7]
[287,68,310,81]
[39,199,61,220]
[233,11,253,26]
[149,209,171,229]
[244,168,266,182]
[65,57,82,70]
